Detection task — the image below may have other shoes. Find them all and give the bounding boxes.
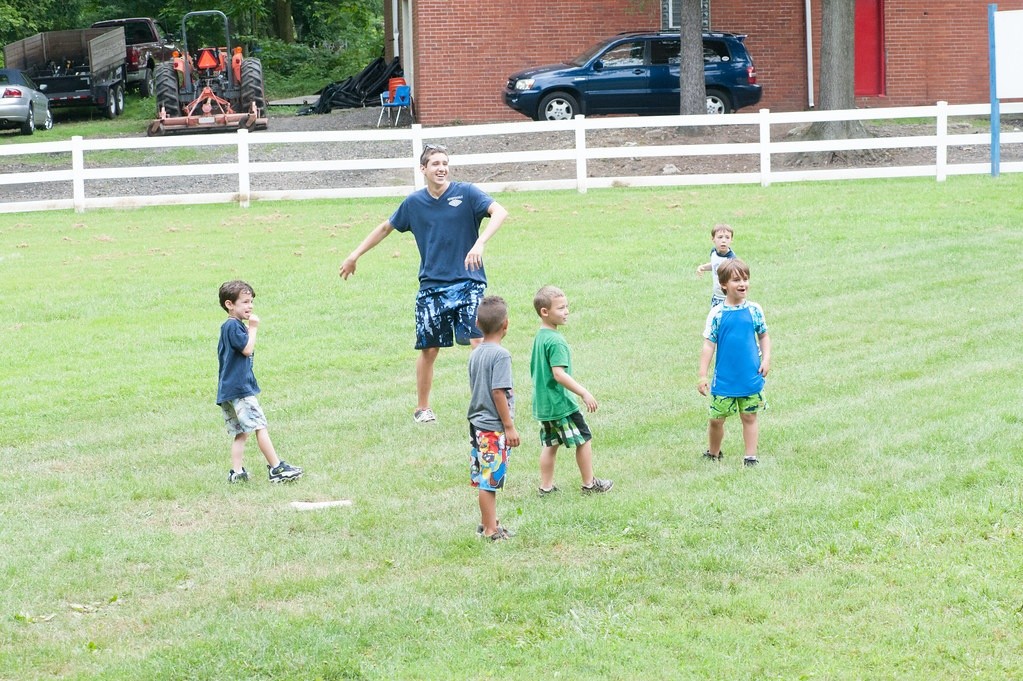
[744,458,759,467]
[704,450,724,462]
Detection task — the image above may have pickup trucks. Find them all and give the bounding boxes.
[91,17,184,99]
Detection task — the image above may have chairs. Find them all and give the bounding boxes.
[376,77,413,128]
[196,48,218,75]
[629,43,642,65]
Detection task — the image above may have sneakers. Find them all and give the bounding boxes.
[539,485,560,498]
[476,520,514,541]
[267,461,305,483]
[228,467,250,484]
[414,408,435,423]
[581,476,614,496]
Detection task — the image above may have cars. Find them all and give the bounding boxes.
[0,68,53,135]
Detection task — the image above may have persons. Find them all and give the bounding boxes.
[697,259,771,466]
[339,145,509,422]
[695,222,737,309]
[217,281,304,485]
[468,296,520,542]
[530,284,615,498]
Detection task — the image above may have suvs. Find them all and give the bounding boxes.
[148,9,268,135]
[500,29,763,122]
